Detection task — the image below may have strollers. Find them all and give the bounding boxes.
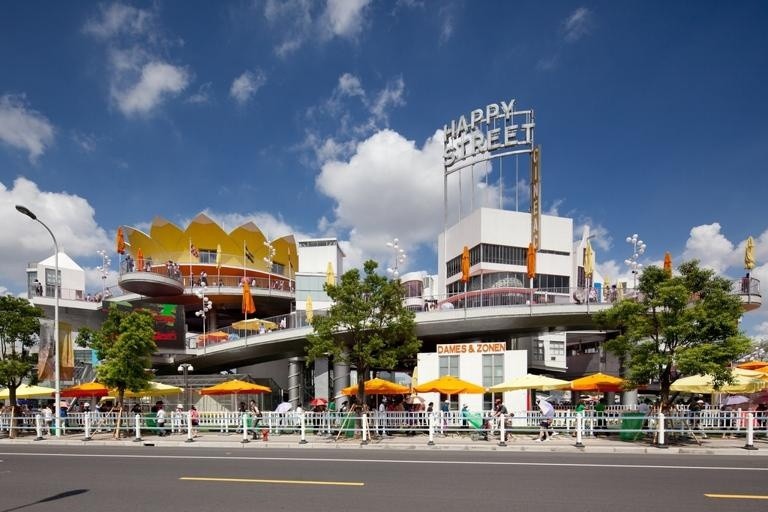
[463,411,490,441]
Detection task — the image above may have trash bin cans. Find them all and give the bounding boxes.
[342,411,356,437]
[51,419,64,436]
[145,413,164,435]
[621,413,647,440]
[243,417,260,434]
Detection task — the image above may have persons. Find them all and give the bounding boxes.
[572,398,705,438]
[33,255,295,303]
[459,399,554,443]
[238,397,449,442]
[1,399,200,442]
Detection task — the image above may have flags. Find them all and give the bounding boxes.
[190,243,295,270]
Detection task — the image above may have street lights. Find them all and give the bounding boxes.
[176,363,193,414]
[384,238,407,281]
[262,239,275,292]
[95,249,111,300]
[14,204,61,438]
[193,280,213,357]
[623,234,646,290]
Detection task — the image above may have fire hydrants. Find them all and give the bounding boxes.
[262,428,269,441]
